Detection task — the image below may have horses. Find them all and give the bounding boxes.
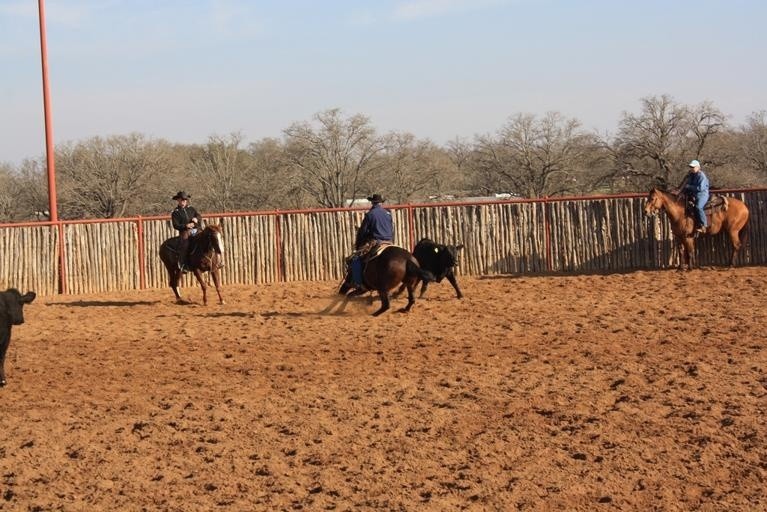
[642,184,752,274]
[320,226,436,318]
[158,217,227,307]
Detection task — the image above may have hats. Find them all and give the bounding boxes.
[689,160,700,167]
[367,194,386,203]
[172,191,191,200]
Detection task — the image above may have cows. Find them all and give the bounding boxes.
[390,237,465,301]
[0,287,36,387]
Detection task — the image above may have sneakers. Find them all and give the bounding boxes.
[696,226,706,234]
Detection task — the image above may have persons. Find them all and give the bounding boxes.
[168,191,204,276]
[668,159,710,236]
[345,194,395,291]
